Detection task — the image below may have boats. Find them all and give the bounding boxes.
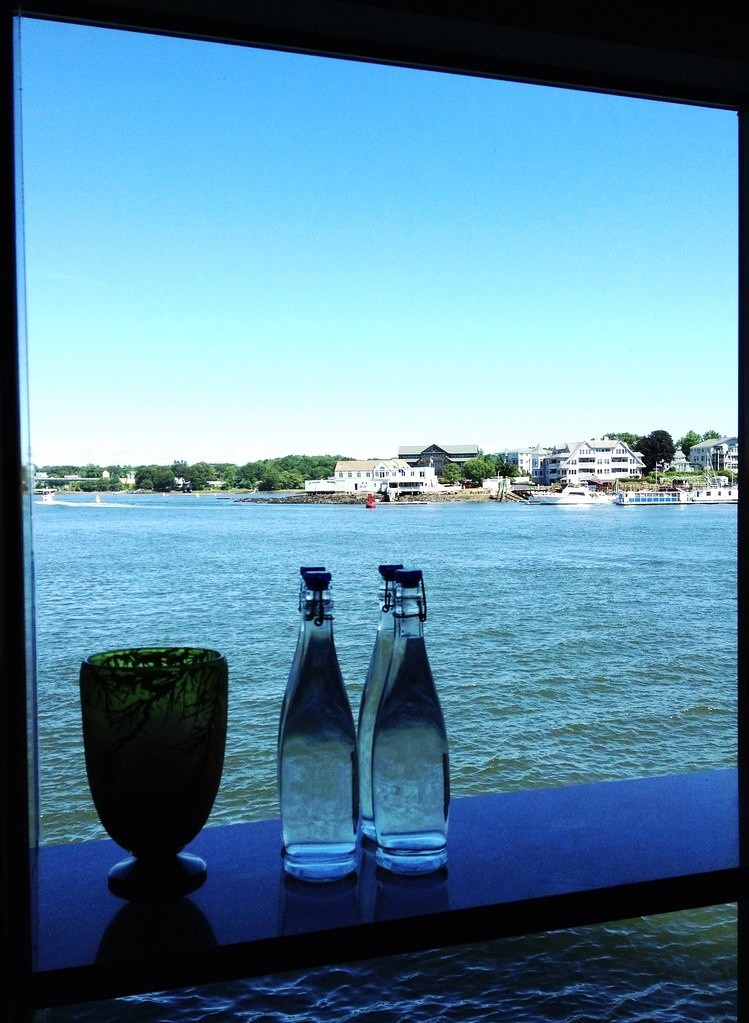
[525,450,738,505]
[43,488,53,502]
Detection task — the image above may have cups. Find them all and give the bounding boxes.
[78,647,230,901]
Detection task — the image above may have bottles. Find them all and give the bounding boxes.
[371,568,451,877]
[281,572,364,883]
[356,564,405,841]
[278,565,330,756]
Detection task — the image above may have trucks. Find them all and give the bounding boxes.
[183,484,192,493]
